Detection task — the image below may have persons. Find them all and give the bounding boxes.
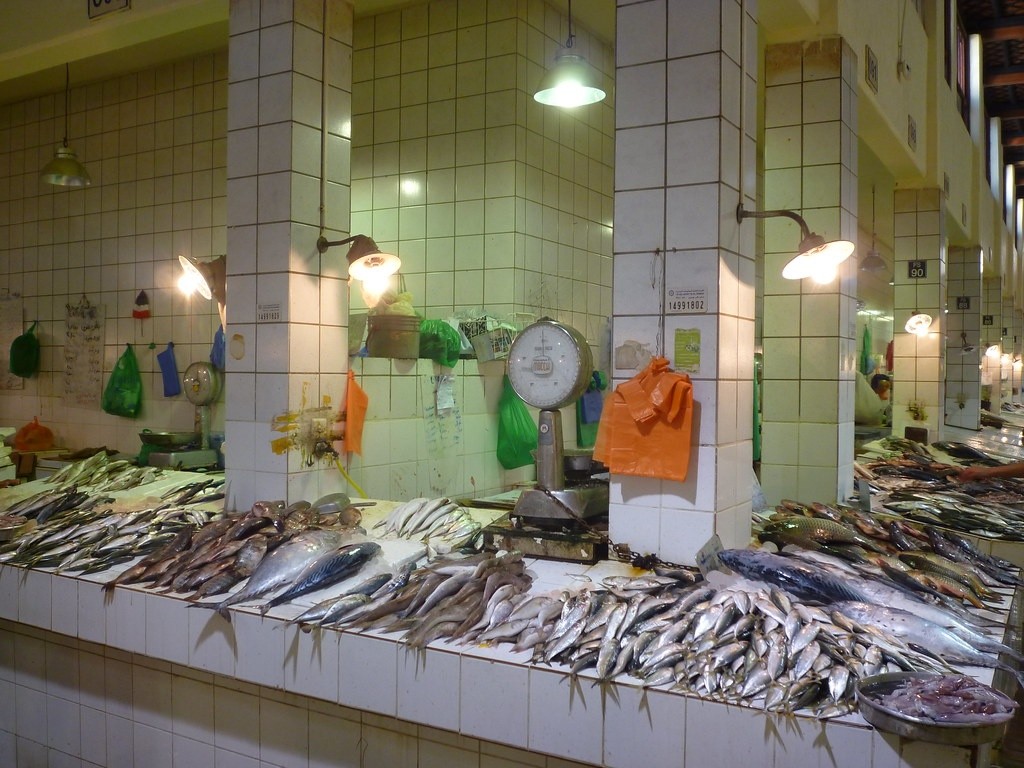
[0,479,20,488]
[870,373,891,423]
[960,462,1024,483]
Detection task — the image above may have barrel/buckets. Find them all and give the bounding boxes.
[365,315,421,360]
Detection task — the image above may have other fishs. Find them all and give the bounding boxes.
[1001,401,1023,414]
[1,434,1023,726]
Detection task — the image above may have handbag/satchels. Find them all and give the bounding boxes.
[101,345,140,418]
[418,319,460,367]
[16,416,53,450]
[497,374,539,469]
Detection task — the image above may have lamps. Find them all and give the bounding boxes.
[317,233,401,290]
[39,87,92,187]
[904,310,934,336]
[737,201,856,287]
[959,332,978,357]
[533,0,607,108]
[177,252,227,301]
[857,188,889,273]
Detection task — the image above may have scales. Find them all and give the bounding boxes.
[506,315,610,521]
[136,361,224,471]
